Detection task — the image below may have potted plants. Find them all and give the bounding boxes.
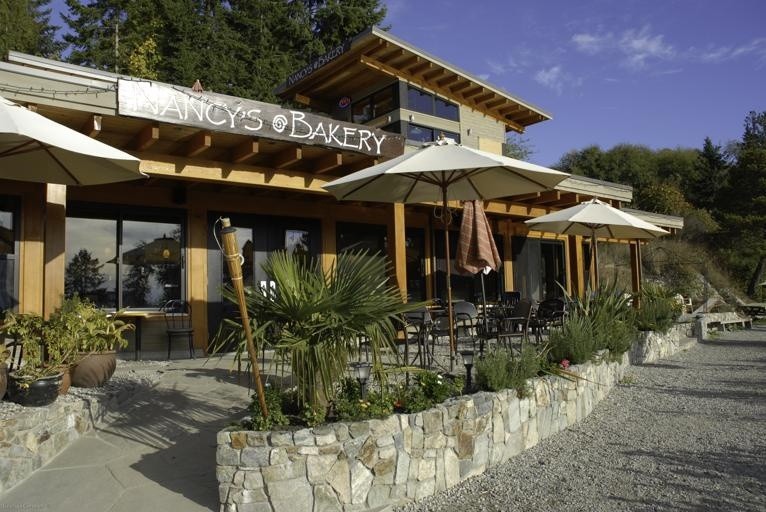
[0,292,137,407]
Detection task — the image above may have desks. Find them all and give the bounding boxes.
[104,315,145,361]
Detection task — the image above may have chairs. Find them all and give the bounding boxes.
[163,300,195,361]
[357,291,569,371]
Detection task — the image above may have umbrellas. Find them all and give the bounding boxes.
[0,95,150,187]
[107,233,180,298]
[0,225,14,255]
[454,200,504,353]
[524,197,671,292]
[321,131,571,372]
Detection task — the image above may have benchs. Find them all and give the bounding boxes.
[711,317,753,331]
